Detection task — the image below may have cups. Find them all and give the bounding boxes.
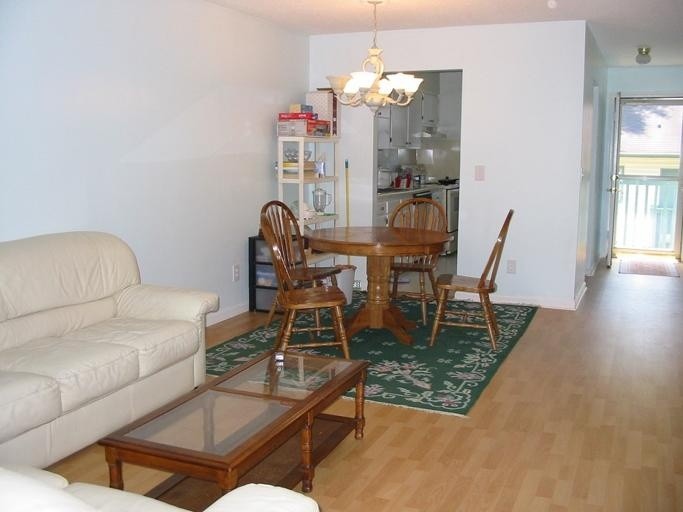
[312,188,333,211]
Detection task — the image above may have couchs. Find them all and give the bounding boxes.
[0,231,220,469]
[0,464,320,512]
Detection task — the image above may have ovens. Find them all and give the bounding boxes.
[443,188,458,256]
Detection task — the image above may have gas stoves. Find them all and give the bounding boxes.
[416,181,459,189]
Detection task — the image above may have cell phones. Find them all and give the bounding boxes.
[274,352,284,362]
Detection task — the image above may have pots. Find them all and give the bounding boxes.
[438,176,459,185]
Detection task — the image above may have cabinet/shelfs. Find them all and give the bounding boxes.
[248,236,306,312]
[377,194,414,265]
[376,92,439,149]
[277,135,340,269]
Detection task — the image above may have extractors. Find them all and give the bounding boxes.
[421,130,447,144]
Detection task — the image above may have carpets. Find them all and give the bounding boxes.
[205,288,538,419]
[618,252,682,278]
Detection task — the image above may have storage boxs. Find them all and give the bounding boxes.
[305,93,338,135]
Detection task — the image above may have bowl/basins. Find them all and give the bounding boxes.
[284,150,312,161]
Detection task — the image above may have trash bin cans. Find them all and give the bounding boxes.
[326,265,357,306]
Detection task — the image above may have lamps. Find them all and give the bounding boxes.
[634,43,650,64]
[327,1,424,113]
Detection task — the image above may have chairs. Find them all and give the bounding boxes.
[431,209,514,351]
[259,198,351,360]
[385,198,448,327]
